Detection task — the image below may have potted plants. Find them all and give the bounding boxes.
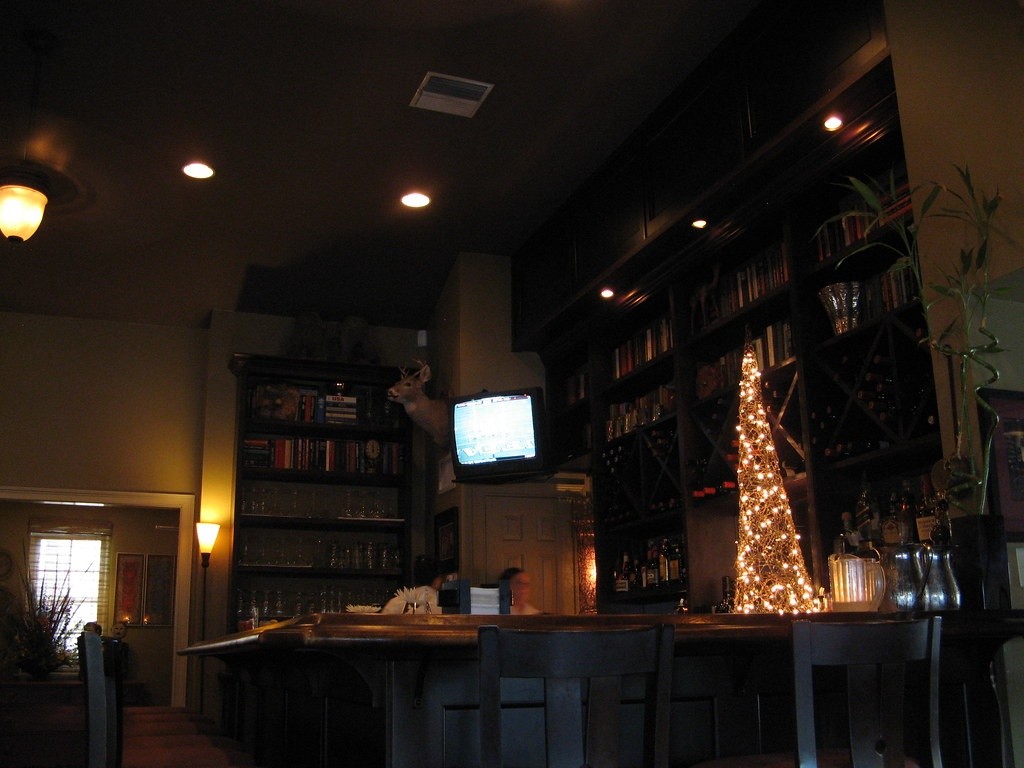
[804,159,1013,605]
[0,535,80,678]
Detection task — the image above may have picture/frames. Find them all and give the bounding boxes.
[112,551,146,626]
[142,553,177,627]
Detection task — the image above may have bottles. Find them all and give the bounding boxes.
[616,539,680,593]
[602,326,940,525]
[721,576,734,613]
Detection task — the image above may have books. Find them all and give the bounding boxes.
[243,437,400,473]
[814,172,923,317]
[684,242,793,387]
[247,385,357,425]
[555,309,678,457]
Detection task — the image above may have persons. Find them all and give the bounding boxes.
[386,555,445,610]
[502,568,541,615]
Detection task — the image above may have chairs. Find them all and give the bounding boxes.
[478,620,675,768]
[691,610,944,768]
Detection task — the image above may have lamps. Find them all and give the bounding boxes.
[195,522,222,714]
[0,163,56,243]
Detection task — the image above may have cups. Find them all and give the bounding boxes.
[818,280,865,335]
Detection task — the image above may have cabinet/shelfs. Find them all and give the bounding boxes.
[539,168,941,623]
[225,351,422,639]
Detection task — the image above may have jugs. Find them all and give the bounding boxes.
[878,543,933,620]
[921,544,960,611]
